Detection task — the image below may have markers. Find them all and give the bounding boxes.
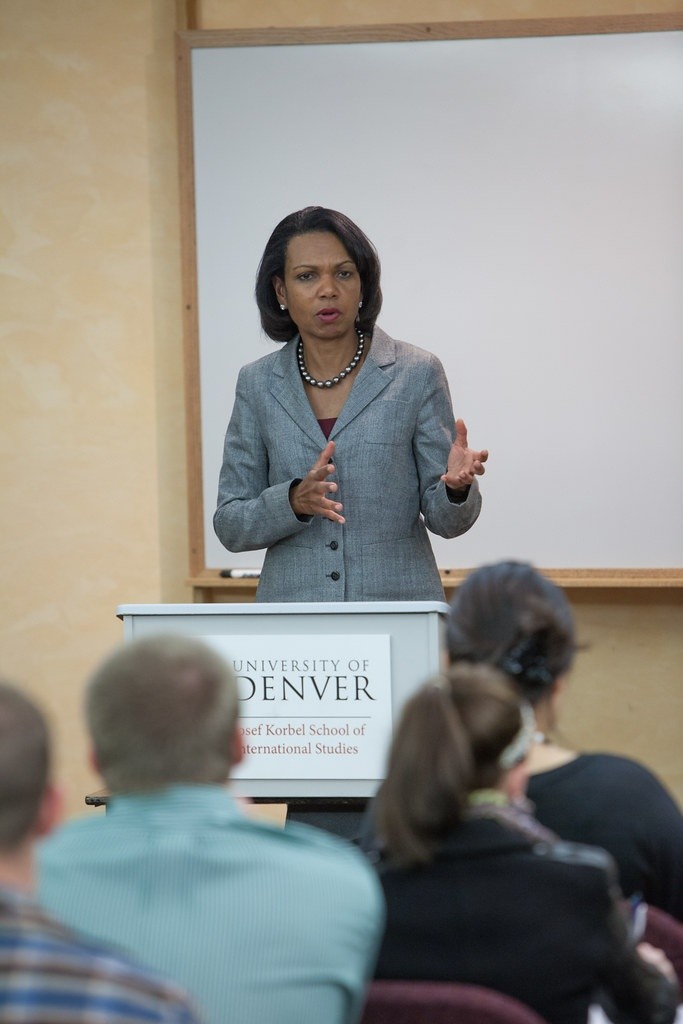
[219,568,262,580]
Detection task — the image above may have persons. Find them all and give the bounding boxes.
[444,561,683,923]
[361,664,679,1024]
[0,684,201,1024]
[213,207,489,604]
[34,634,385,1024]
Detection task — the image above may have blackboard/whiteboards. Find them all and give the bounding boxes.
[174,13,683,597]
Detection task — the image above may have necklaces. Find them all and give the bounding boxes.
[298,330,365,387]
